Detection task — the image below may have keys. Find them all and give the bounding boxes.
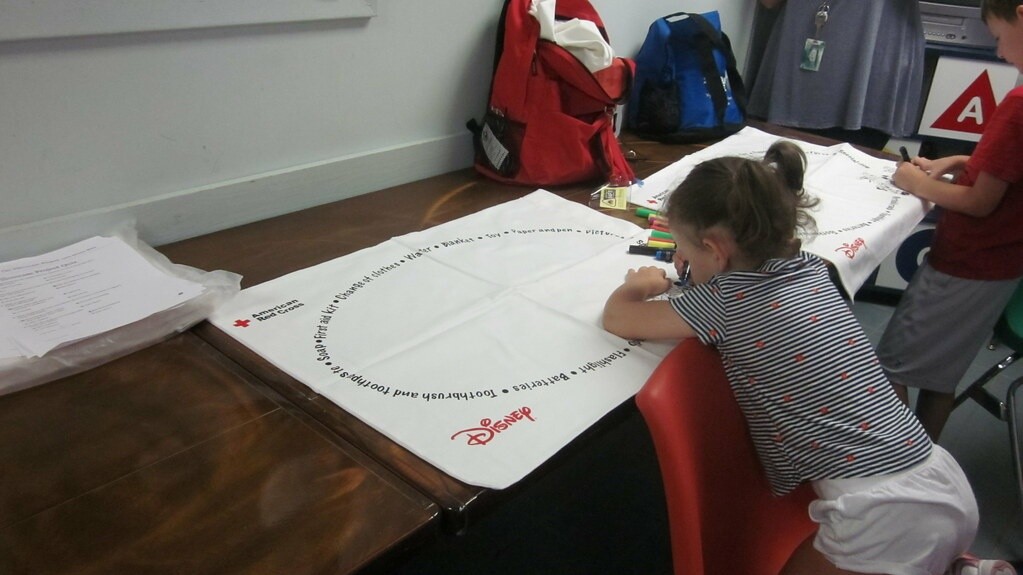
[812,1,831,40]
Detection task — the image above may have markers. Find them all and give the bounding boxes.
[628,205,680,262]
[900,147,913,166]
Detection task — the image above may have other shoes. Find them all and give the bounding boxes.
[978,559,1021,575]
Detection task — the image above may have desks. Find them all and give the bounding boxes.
[140,117,952,515]
[0,257,441,575]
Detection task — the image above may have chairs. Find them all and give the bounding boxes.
[635,333,825,574]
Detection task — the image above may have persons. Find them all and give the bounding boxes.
[599,137,1022,574]
[870,0,1023,446]
[745,0,927,151]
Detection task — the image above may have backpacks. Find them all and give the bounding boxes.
[465,0,636,188]
[633,11,745,141]
